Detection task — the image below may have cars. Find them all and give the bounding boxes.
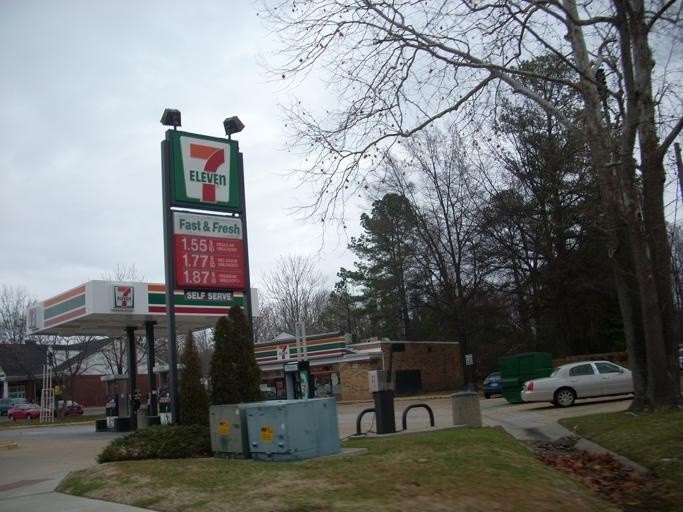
[481,370,502,400]
[520,360,635,408]
[56,398,84,417]
[0,397,28,416]
[678,347,682,371]
[7,404,51,422]
